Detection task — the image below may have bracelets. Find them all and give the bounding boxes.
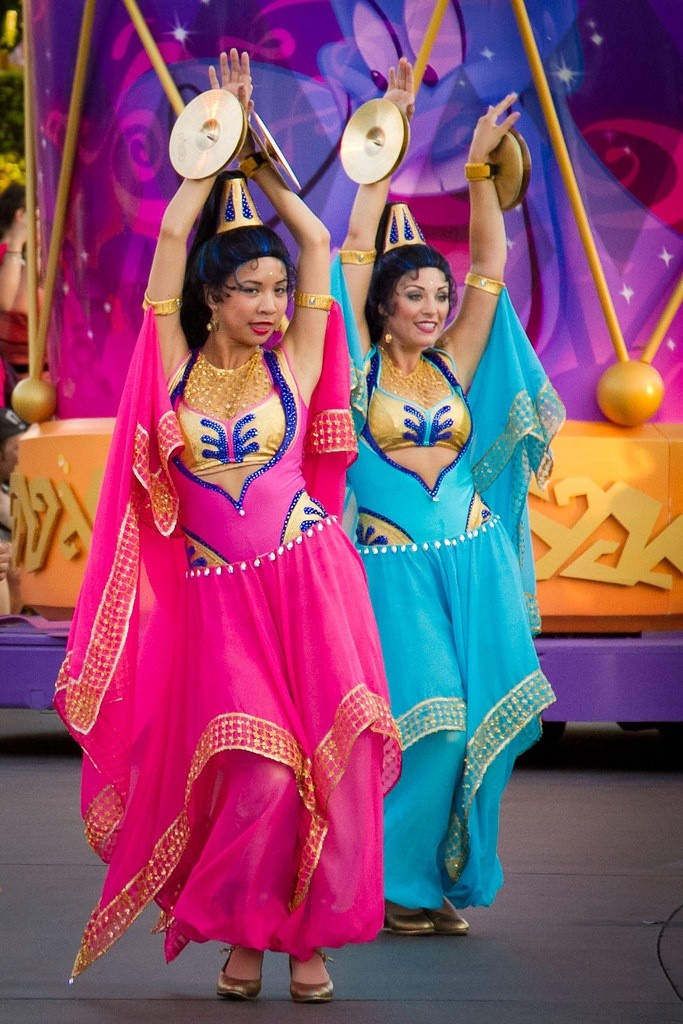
[238,151,272,180]
[464,162,498,181]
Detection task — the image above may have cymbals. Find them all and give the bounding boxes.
[339,97,412,185]
[169,88,248,181]
[248,108,301,192]
[485,126,533,210]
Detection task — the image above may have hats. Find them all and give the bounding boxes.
[0,408,29,441]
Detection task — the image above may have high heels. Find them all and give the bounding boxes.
[384,910,435,935]
[216,946,263,999]
[423,908,469,933]
[289,951,333,1001]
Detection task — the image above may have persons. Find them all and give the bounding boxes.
[50,45,403,1005]
[328,56,567,937]
